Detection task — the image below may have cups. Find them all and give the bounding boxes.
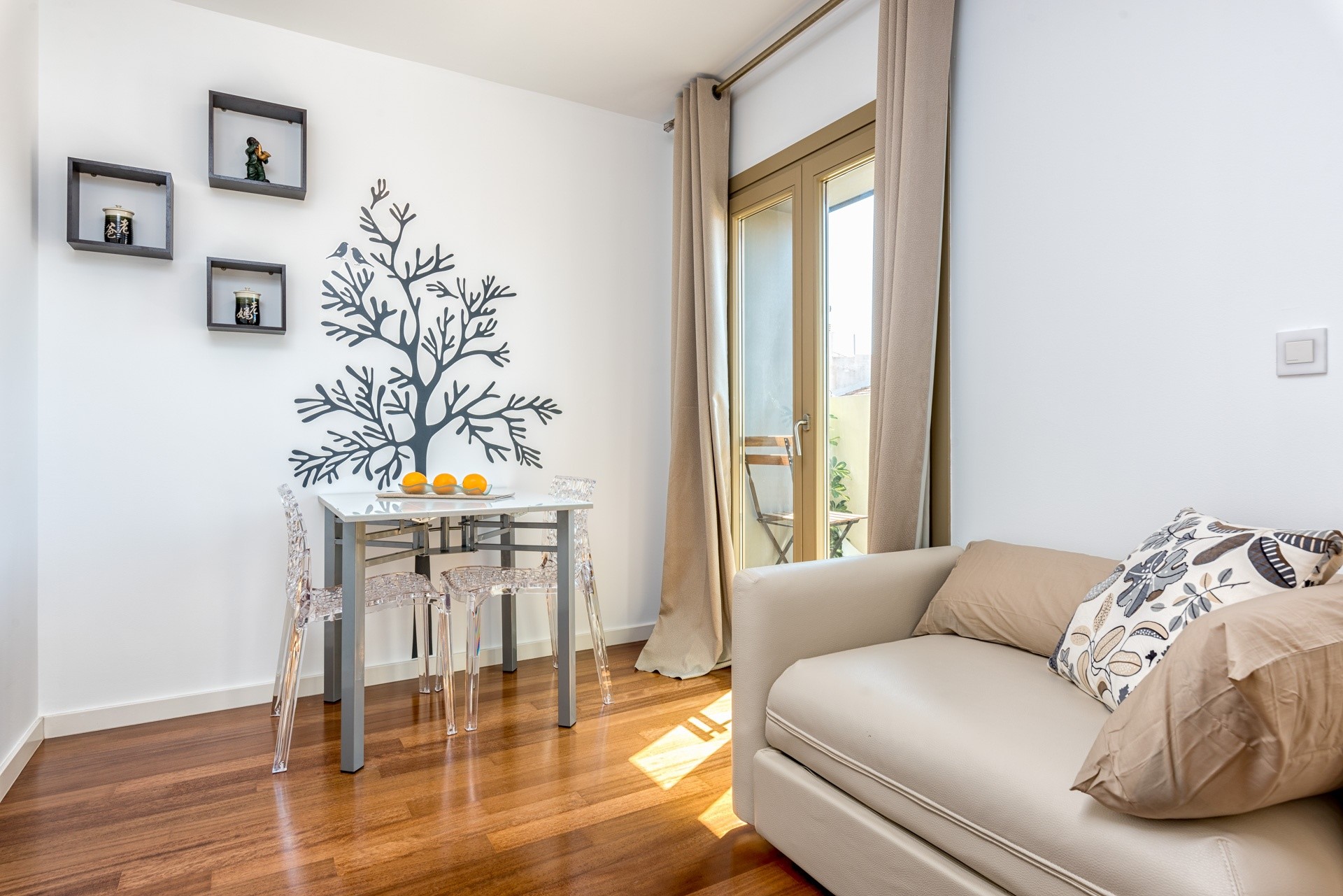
[103,205,135,244]
[233,287,261,325]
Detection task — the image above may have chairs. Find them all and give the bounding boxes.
[435,476,615,731]
[744,434,869,565]
[269,483,457,774]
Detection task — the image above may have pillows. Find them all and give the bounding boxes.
[910,538,1121,655]
[1047,505,1343,712]
[1071,581,1343,820]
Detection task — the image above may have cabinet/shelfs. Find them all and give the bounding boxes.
[206,256,286,336]
[66,155,174,261]
[207,89,308,202]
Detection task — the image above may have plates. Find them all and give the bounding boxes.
[398,484,492,495]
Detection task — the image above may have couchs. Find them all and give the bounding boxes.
[731,540,1343,896]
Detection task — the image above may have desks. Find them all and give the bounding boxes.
[317,488,592,775]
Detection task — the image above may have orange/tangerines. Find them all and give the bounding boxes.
[402,472,427,494]
[463,474,487,495]
[433,474,457,495]
